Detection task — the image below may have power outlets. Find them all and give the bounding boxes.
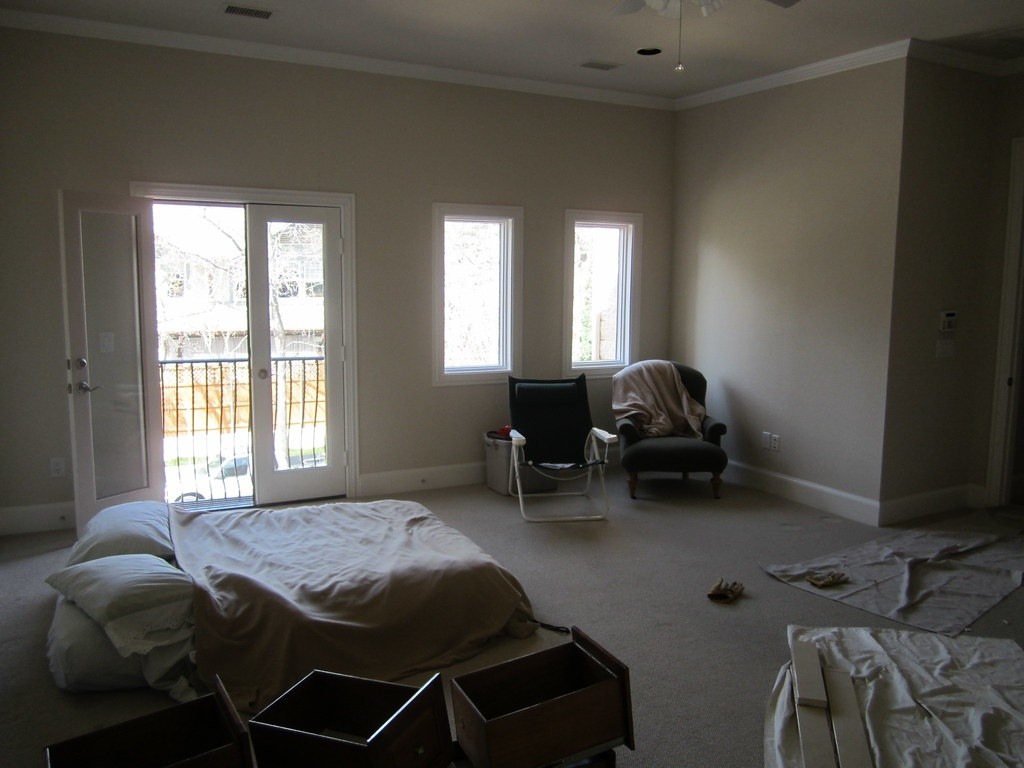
[771,435,779,450]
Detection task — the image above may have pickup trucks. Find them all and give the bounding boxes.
[169,448,326,502]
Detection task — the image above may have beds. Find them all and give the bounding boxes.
[47,499,539,700]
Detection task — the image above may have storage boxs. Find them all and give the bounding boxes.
[482,432,559,496]
[44,674,253,768]
[248,667,455,767]
[449,627,634,767]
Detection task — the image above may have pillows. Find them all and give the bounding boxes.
[45,554,197,658]
[67,500,175,570]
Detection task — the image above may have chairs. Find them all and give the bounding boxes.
[507,375,619,522]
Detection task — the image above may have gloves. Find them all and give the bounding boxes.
[707,578,744,603]
[805,572,849,589]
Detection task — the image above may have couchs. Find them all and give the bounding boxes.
[611,360,727,499]
